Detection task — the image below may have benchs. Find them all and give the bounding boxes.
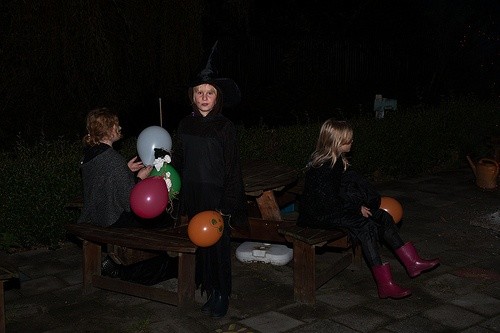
[0,256,19,333]
[278,212,352,303]
[60,219,200,307]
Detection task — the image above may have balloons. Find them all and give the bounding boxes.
[150,163,182,200]
[129,175,169,219]
[378,197,403,224]
[187,210,224,247]
[136,125,172,170]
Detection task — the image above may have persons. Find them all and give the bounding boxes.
[296,118,440,298]
[77,108,153,278]
[167,74,240,316]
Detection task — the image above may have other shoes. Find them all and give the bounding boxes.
[102,254,121,281]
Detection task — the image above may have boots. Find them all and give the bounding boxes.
[371,262,415,299]
[395,240,440,277]
[201,286,229,317]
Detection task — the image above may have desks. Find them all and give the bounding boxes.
[237,158,299,244]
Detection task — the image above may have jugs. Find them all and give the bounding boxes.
[466,155,499,191]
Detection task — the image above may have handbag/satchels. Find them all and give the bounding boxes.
[129,254,178,285]
